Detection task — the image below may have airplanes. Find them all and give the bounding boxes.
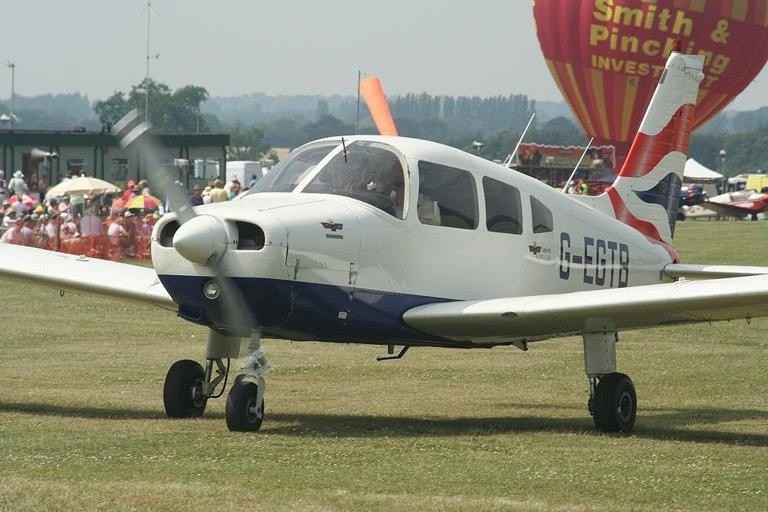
[0,52,768,431]
[683,189,767,221]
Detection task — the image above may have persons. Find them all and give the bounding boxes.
[0,169,259,262]
[577,178,587,195]
[367,159,441,224]
[568,180,578,194]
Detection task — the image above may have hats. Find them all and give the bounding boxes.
[193,173,239,189]
[3,170,149,221]
